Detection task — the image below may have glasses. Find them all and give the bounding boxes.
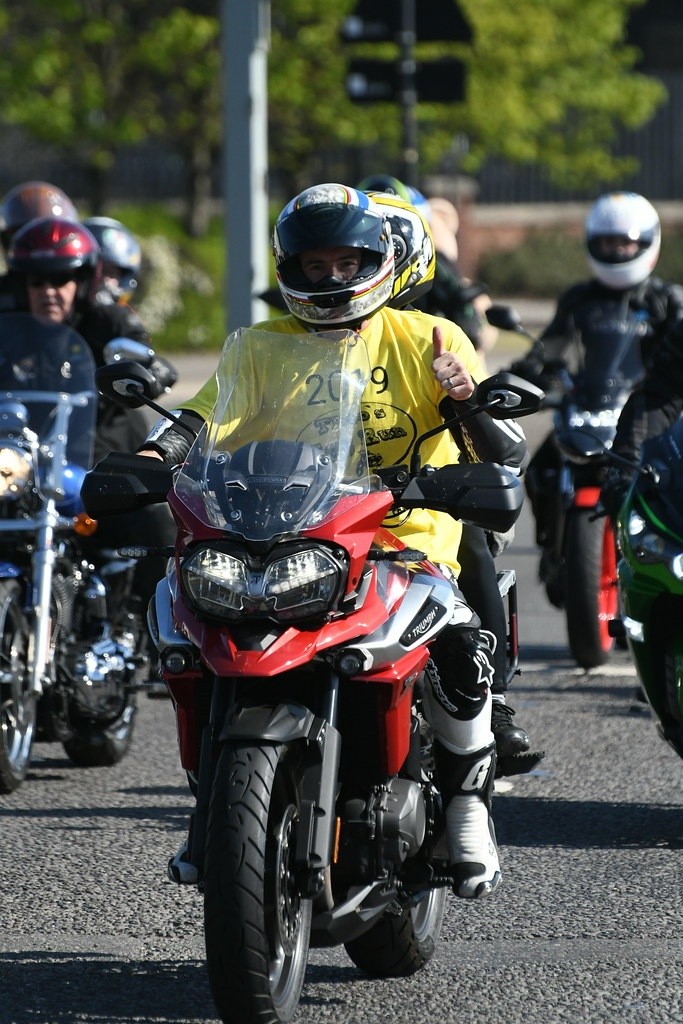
[26,274,77,290]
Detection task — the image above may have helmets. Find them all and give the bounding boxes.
[6,216,103,305]
[356,190,436,310]
[0,181,80,249]
[82,216,142,304]
[358,173,462,262]
[272,183,393,328]
[583,191,661,289]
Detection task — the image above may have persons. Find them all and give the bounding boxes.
[2,216,179,691]
[136,182,531,899]
[509,189,683,583]
[1,182,85,295]
[340,174,499,360]
[68,214,147,312]
[595,319,683,551]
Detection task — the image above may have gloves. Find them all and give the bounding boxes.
[599,459,635,515]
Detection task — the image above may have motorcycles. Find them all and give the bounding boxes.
[97,326,544,1024]
[0,314,138,796]
[549,402,681,760]
[483,305,647,669]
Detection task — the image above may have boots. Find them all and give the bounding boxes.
[425,661,504,900]
[166,770,212,886]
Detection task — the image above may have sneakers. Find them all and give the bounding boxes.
[490,699,532,752]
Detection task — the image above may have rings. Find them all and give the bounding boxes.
[446,377,454,389]
